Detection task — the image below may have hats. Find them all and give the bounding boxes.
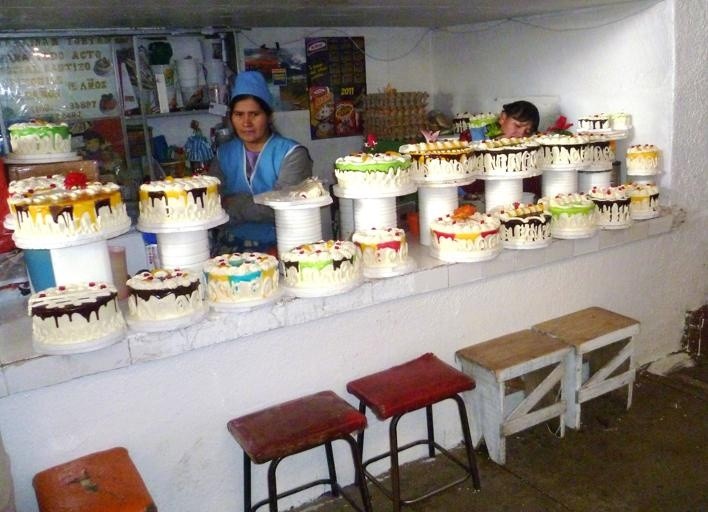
[231,72,273,112]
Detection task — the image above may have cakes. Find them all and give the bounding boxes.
[126,268,203,320]
[430,205,502,252]
[400,130,474,177]
[621,182,659,211]
[27,283,121,344]
[8,119,72,155]
[138,174,223,222]
[94,56,114,74]
[204,251,279,302]
[7,175,131,240]
[624,144,658,170]
[470,137,539,175]
[540,113,632,168]
[537,192,596,232]
[335,136,413,188]
[584,185,632,225]
[492,202,552,243]
[280,240,362,287]
[353,227,408,267]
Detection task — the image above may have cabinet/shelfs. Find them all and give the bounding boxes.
[107,23,249,198]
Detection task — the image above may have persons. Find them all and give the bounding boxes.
[204,71,314,259]
[79,130,101,160]
[457,101,540,200]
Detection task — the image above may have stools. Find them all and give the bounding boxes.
[226,390,371,510]
[346,353,482,512]
[31,447,158,510]
[454,306,642,466]
